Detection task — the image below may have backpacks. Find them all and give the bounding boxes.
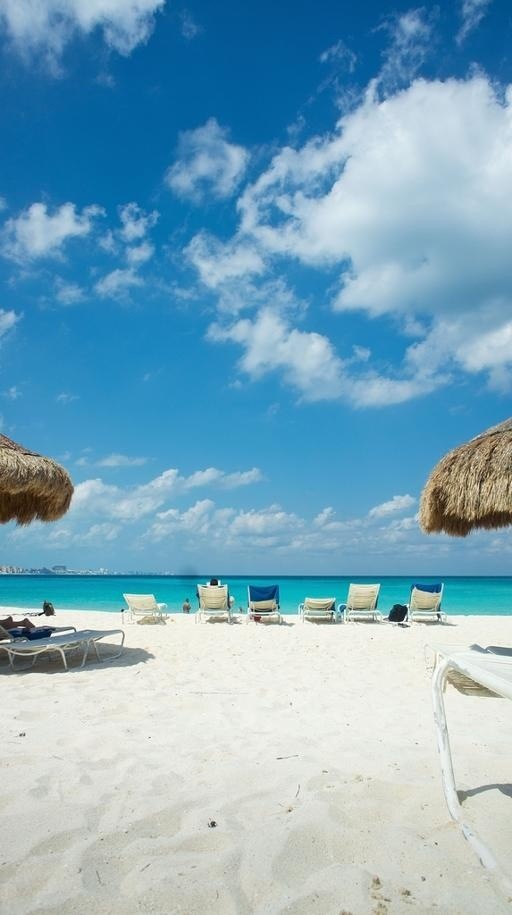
[389,604,408,622]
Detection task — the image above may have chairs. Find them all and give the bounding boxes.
[299,598,338,625]
[403,583,448,625]
[0,624,125,670]
[244,585,283,626]
[338,582,383,626]
[196,583,242,624]
[120,593,167,627]
[422,641,511,911]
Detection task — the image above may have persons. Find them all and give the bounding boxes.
[183,597,191,614]
[196,579,235,610]
[0,616,35,630]
